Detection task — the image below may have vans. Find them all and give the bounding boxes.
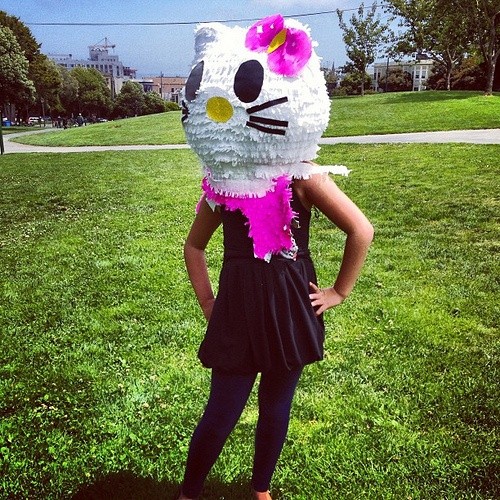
[27,117,45,126]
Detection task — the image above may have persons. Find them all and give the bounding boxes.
[37,109,98,130]
[175,17,377,500]
